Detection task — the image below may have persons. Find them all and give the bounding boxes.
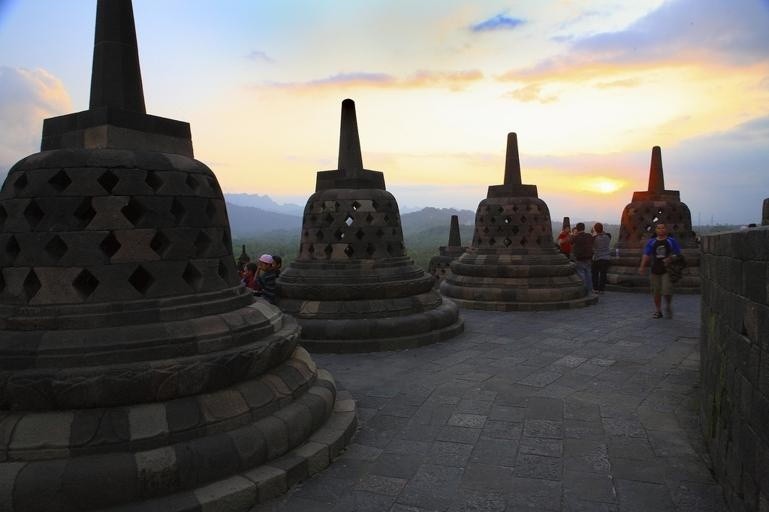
[272,255,282,278]
[591,222,612,293]
[568,222,594,294]
[572,227,579,257]
[558,225,571,257]
[255,253,278,305]
[638,221,686,319]
[241,261,261,290]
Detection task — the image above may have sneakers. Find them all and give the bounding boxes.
[592,288,607,295]
[651,312,663,319]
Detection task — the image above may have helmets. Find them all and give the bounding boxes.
[258,254,273,264]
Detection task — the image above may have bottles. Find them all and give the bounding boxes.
[616,249,619,258]
